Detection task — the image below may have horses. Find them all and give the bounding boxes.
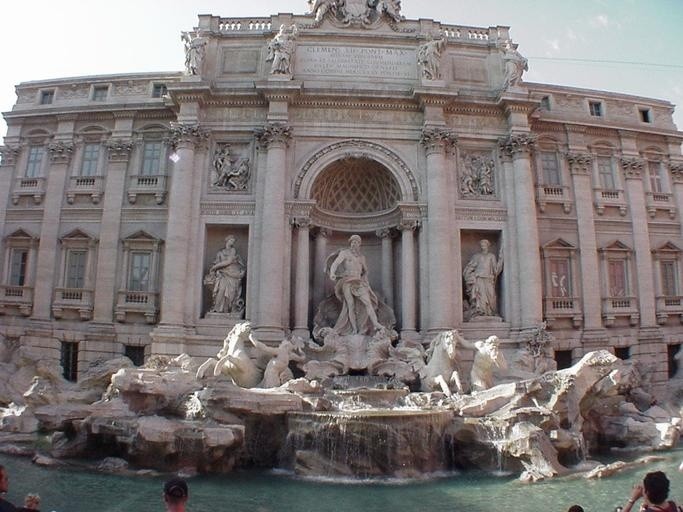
[195,321,264,388]
[419,329,463,397]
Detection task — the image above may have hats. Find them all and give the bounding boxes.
[164,478,188,498]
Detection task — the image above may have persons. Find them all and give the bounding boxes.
[507,48,528,86]
[463,239,504,316]
[250,332,305,388]
[419,32,448,80]
[216,148,249,190]
[186,28,208,76]
[0,465,41,512]
[462,157,493,195]
[162,476,189,512]
[457,334,508,393]
[329,234,383,335]
[209,234,246,312]
[568,505,584,512]
[621,470,682,512]
[268,24,297,73]
